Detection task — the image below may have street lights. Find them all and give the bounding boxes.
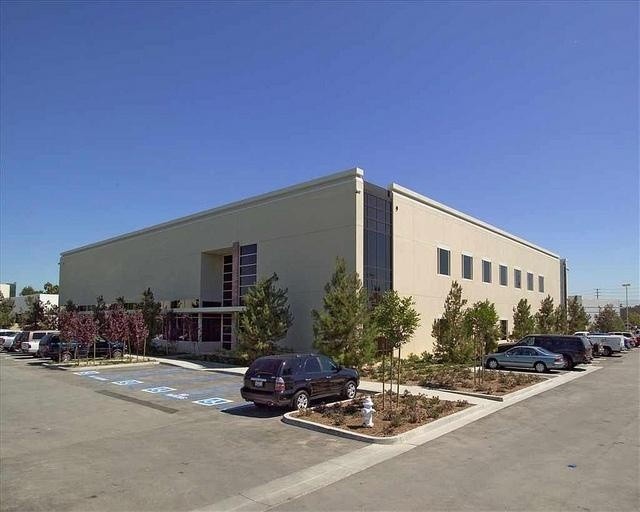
[622,283,631,330]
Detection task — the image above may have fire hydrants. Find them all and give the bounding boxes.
[360,394,375,429]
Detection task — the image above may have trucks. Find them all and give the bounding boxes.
[572,330,624,357]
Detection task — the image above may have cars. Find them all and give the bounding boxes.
[607,329,640,349]
[481,345,568,373]
[0,329,60,358]
[624,323,638,329]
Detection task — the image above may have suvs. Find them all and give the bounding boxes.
[38,332,128,363]
[239,352,361,411]
[497,332,594,371]
